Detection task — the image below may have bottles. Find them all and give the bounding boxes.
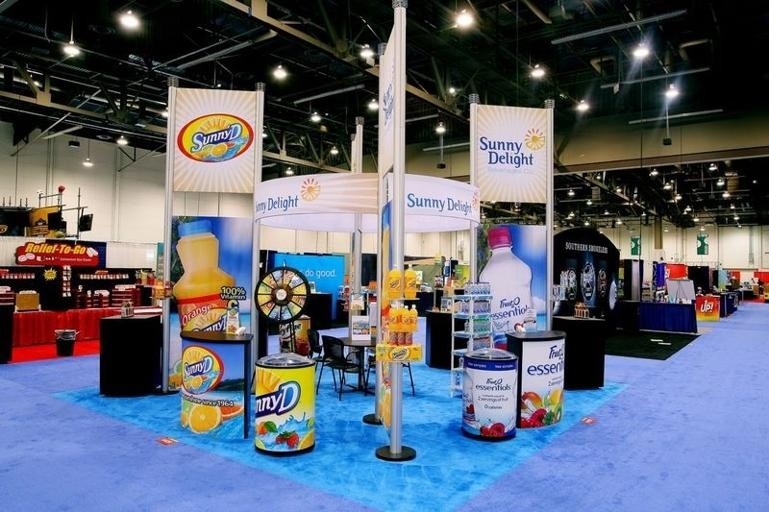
[120,300,136,317]
[380,207,391,342]
[387,302,418,331]
[171,219,235,333]
[574,302,590,319]
[478,225,537,352]
[387,265,402,298]
[455,281,491,386]
[404,264,417,298]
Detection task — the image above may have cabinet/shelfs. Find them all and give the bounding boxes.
[449,294,494,398]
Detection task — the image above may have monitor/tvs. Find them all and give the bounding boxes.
[80,213,93,231]
[48,212,61,230]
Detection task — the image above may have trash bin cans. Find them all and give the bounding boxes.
[54,329,76,356]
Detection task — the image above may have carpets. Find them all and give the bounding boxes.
[8,338,100,364]
[50,357,630,512]
[604,328,703,362]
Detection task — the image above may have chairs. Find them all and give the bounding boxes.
[306,327,416,401]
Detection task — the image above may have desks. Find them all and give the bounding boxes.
[552,315,606,389]
[640,301,698,333]
[12,305,159,349]
[99,314,159,399]
